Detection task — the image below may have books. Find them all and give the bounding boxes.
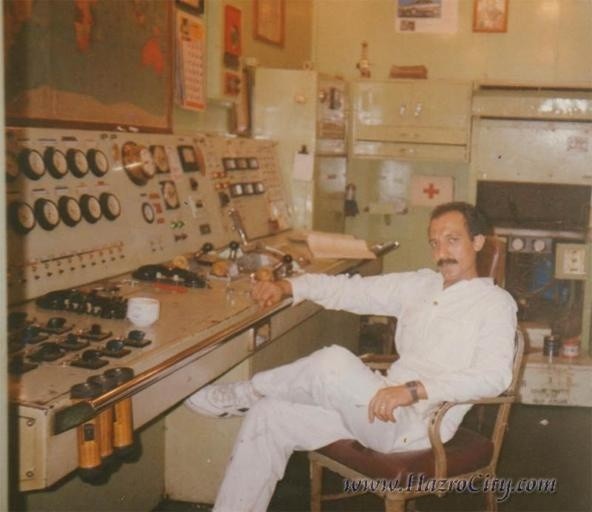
[304,229,378,260]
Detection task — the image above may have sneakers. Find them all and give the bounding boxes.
[184,380,261,417]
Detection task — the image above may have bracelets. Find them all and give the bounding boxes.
[406,381,419,402]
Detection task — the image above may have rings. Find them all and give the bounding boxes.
[379,405,386,409]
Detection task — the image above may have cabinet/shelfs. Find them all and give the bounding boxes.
[346,76,473,162]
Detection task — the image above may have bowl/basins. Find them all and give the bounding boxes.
[128,297,161,329]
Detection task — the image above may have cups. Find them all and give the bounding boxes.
[563,338,580,357]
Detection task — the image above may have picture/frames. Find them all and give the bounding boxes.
[252,0,285,46]
[472,0,508,33]
[176,0,205,15]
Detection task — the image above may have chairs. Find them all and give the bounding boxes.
[307,236,530,512]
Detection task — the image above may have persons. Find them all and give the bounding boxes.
[186,202,518,511]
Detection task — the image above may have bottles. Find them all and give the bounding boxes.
[358,41,369,77]
[542,335,560,356]
